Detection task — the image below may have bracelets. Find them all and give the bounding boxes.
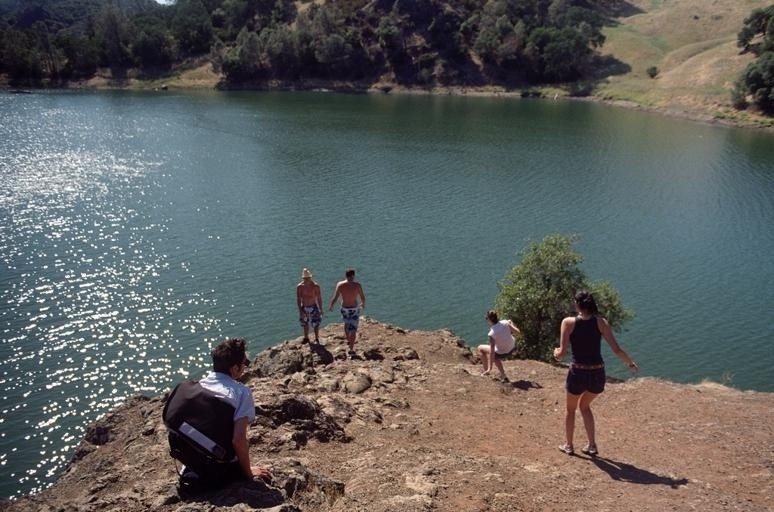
[628,362,636,367]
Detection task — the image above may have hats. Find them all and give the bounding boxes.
[302,268,312,278]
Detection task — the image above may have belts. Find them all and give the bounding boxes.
[570,362,604,369]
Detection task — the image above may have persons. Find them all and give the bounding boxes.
[329,269,366,355]
[297,269,324,344]
[553,291,640,456]
[168,338,272,483]
[477,310,520,383]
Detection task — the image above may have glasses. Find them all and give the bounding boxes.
[242,359,251,367]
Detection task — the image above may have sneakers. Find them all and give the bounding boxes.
[314,339,319,344]
[348,350,356,354]
[559,444,573,454]
[302,338,309,344]
[583,445,598,454]
